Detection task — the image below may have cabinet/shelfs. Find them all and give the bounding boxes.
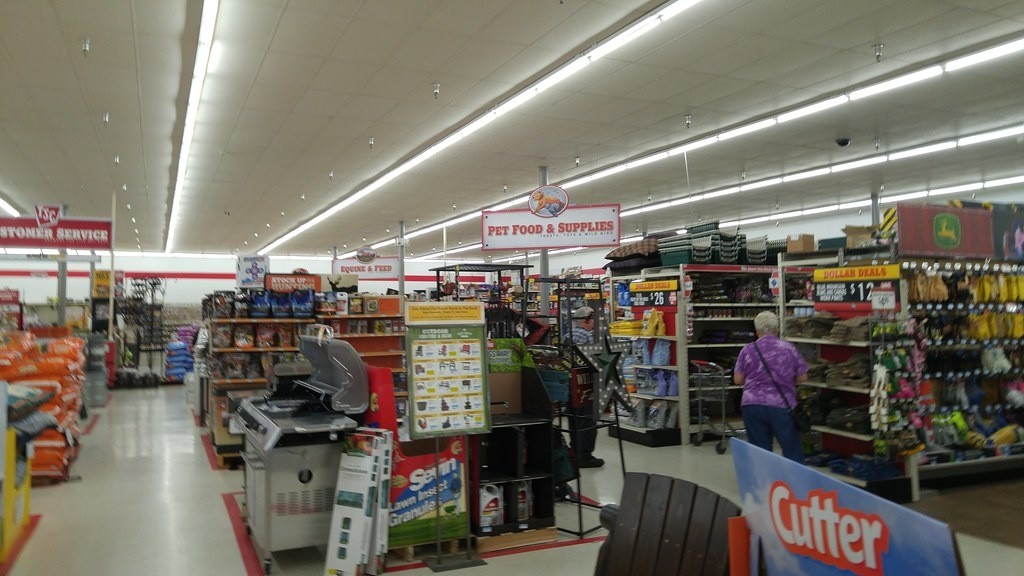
[138,249,1024,562]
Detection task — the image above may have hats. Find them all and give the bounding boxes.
[574,306,594,320]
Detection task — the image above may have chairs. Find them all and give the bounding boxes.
[604,472,742,576]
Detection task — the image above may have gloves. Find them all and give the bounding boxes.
[914,268,1024,450]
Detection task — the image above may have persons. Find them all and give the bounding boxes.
[734,311,809,466]
[563,306,605,468]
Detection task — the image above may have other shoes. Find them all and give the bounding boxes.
[577,455,605,468]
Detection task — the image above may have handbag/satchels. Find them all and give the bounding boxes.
[789,402,810,435]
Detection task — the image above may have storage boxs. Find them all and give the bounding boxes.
[324,427,393,576]
[840,225,881,248]
[489,370,522,416]
[786,233,815,253]
